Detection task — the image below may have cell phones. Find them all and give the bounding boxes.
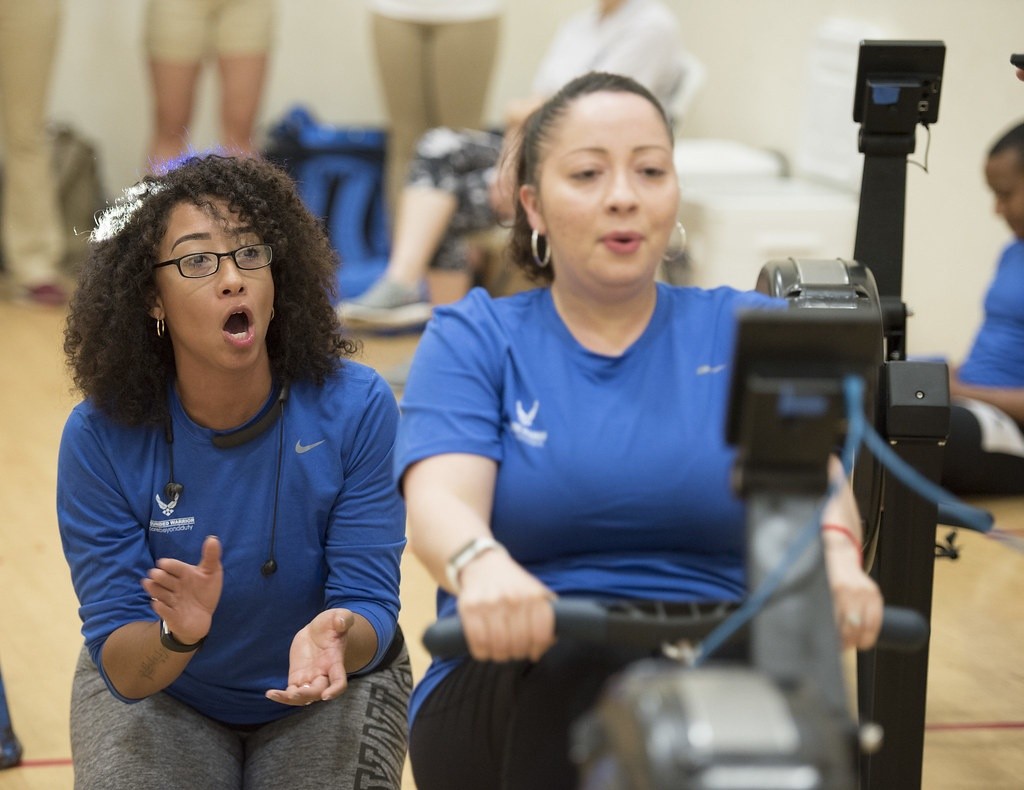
[1009,53,1024,70]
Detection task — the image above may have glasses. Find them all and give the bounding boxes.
[151,243,276,279]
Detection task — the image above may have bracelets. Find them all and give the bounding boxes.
[820,524,863,559]
[160,616,209,652]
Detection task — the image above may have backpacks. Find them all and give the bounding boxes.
[261,108,389,304]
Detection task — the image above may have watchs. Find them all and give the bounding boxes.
[447,538,509,597]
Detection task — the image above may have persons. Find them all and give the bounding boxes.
[0,0,709,332]
[56,150,413,790]
[948,119,1024,494]
[390,71,884,790]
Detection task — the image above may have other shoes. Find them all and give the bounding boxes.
[335,272,435,331]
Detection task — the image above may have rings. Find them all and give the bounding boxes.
[841,613,866,632]
